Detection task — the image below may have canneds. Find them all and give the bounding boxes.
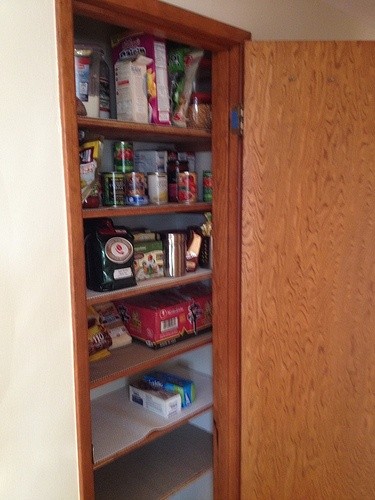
[187,90,213,131]
[102,141,212,208]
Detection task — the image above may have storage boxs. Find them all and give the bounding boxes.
[130,229,159,242]
[132,241,164,282]
[129,382,180,418]
[113,283,213,348]
[135,151,168,190]
[110,31,171,126]
[164,148,196,201]
[145,372,194,408]
[114,55,153,125]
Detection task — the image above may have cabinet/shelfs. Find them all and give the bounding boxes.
[55,1,375,499]
